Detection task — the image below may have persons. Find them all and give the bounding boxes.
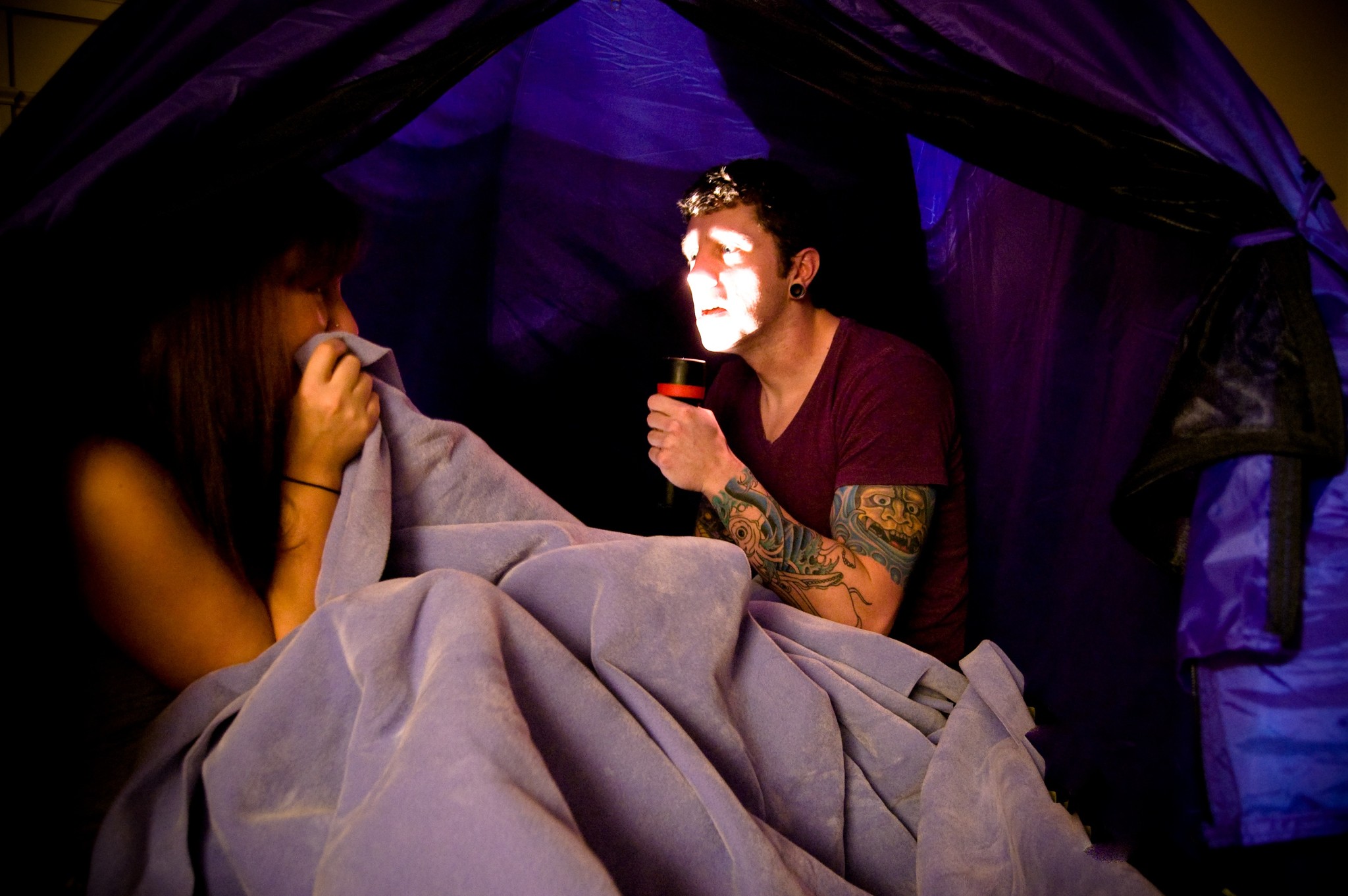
[646,157,969,672]
[0,149,381,896]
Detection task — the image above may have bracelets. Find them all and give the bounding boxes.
[283,477,341,495]
